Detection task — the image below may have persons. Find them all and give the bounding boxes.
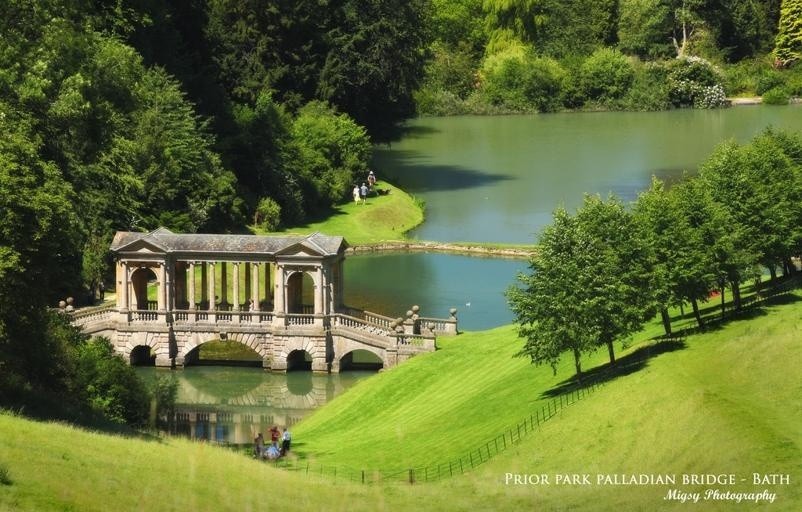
[90,279,105,302]
[353,171,393,204]
[203,295,254,321]
[253,424,291,460]
